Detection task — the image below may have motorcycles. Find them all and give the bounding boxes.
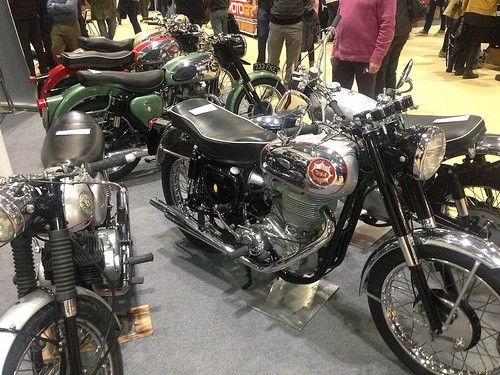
[27,14,500,375]
[0,109,157,375]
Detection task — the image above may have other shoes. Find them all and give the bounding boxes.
[455,70,463,75]
[495,75,500,80]
[463,72,479,78]
[446,68,452,72]
[439,51,448,58]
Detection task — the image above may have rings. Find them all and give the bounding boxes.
[376,70,378,71]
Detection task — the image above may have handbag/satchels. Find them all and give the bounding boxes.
[226,13,239,33]
[451,16,464,41]
[202,8,212,24]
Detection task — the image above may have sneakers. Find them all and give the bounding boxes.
[415,30,428,35]
[434,30,445,36]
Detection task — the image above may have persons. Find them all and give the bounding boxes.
[331,0,397,100]
[375,0,412,102]
[8,0,231,84]
[251,0,339,99]
[415,0,449,36]
[438,0,500,81]
